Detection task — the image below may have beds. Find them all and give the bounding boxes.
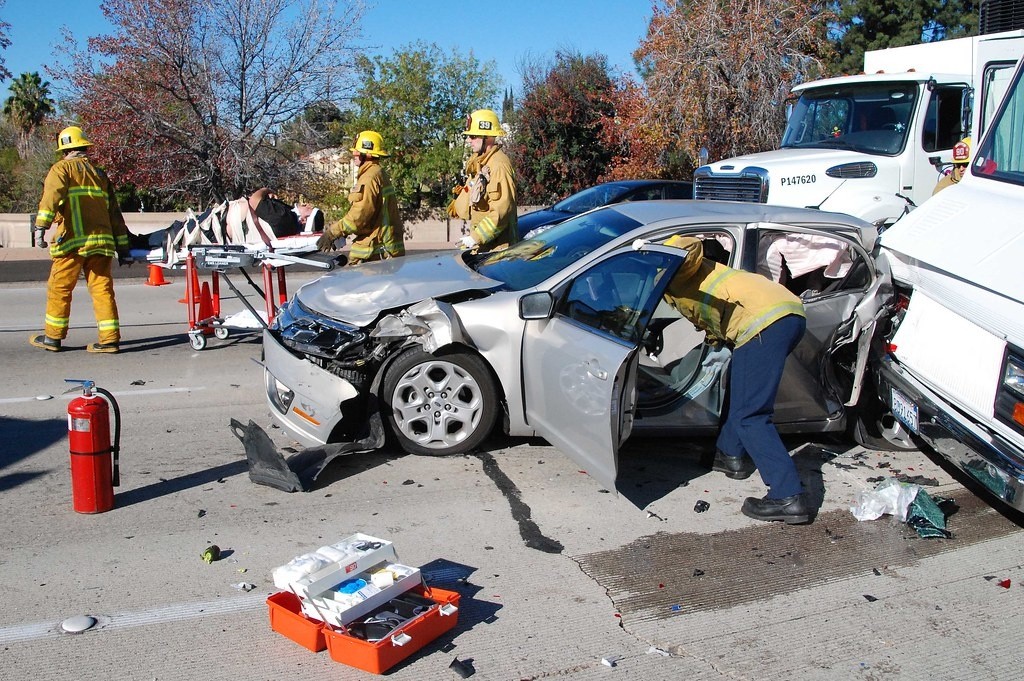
[115,233,346,350]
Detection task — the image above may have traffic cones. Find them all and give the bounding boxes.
[177,253,214,303]
[143,261,172,286]
[196,282,221,336]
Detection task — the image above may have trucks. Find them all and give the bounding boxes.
[693,27,1024,236]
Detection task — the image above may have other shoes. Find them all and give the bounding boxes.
[87,342,119,352]
[30,333,62,350]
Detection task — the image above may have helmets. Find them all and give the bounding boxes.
[949,136,972,163]
[349,131,391,159]
[462,110,508,136]
[55,126,95,151]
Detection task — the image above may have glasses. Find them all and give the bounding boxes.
[469,135,476,139]
[954,163,971,168]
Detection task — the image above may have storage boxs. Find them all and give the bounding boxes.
[266,532,461,675]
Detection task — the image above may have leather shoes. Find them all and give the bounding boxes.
[741,495,808,523]
[700,450,747,479]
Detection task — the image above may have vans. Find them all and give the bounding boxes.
[873,26,1024,519]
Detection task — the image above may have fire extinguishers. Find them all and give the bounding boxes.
[61,378,121,514]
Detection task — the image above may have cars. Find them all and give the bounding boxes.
[517,179,690,242]
[260,198,922,502]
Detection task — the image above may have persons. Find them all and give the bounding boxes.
[448,109,518,251]
[116,186,324,259]
[932,136,971,196]
[632,234,809,526]
[315,130,406,264]
[30,126,132,353]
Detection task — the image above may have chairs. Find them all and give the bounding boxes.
[633,318,705,393]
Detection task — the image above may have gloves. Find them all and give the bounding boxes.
[702,344,732,375]
[117,251,134,267]
[34,226,48,248]
[631,238,651,251]
[316,230,335,253]
[454,236,475,250]
